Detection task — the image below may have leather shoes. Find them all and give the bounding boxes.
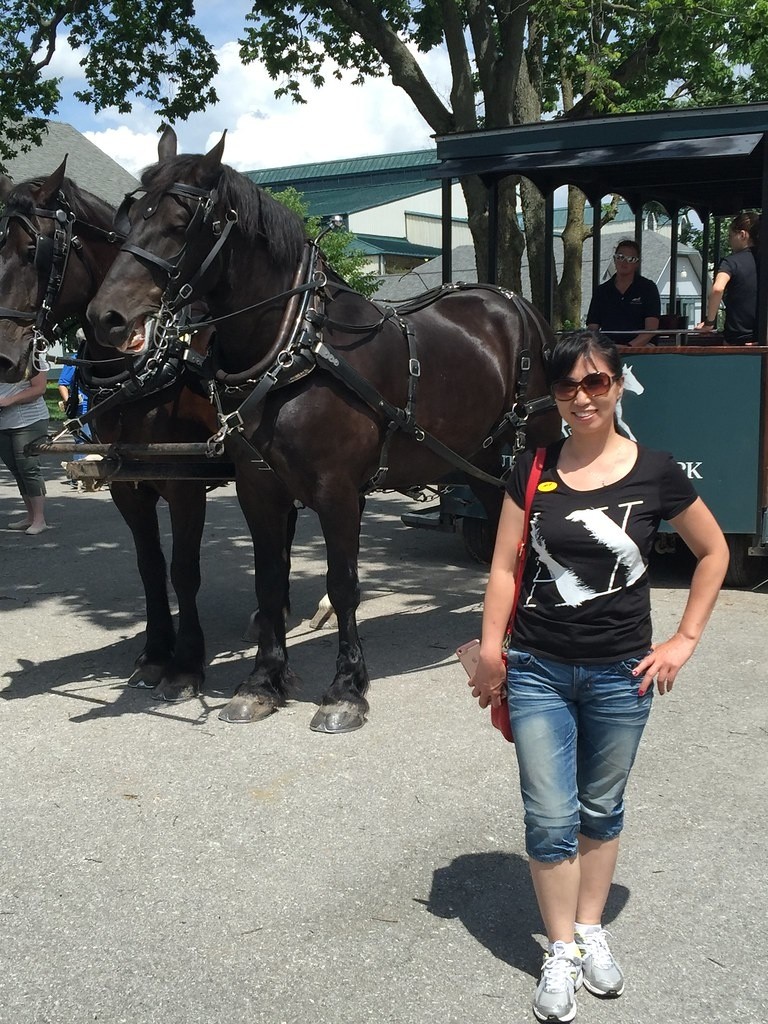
[8,519,49,535]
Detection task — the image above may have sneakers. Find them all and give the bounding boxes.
[71,481,88,489]
[530,922,625,1024]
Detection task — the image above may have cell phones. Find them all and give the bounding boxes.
[457,639,507,705]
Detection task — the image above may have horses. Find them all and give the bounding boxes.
[0,127,562,737]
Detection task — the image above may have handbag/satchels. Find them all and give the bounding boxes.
[492,651,515,744]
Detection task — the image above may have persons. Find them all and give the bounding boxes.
[0,355,50,535]
[695,212,768,346]
[586,240,661,346]
[468,328,729,1024]
[58,327,93,488]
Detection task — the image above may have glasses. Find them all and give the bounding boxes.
[613,253,640,264]
[551,372,622,401]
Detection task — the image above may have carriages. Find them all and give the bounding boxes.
[0,107,768,738]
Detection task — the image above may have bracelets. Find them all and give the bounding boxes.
[705,319,715,326]
